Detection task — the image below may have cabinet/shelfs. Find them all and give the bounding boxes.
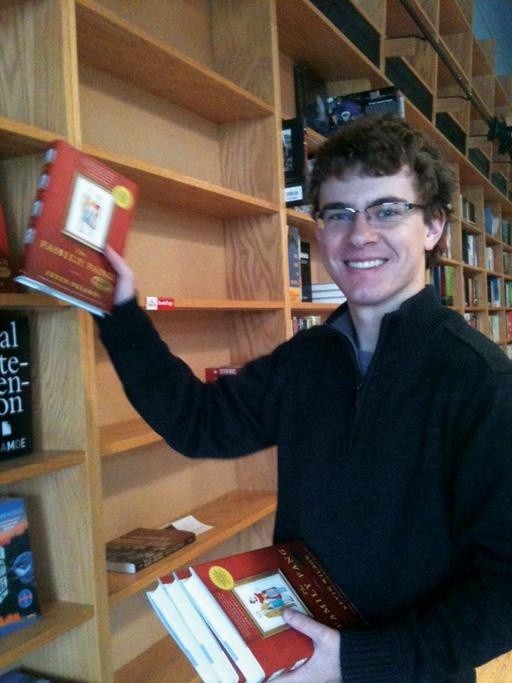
[0,2,511,683]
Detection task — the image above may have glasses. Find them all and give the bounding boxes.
[313,201,425,230]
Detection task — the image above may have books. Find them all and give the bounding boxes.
[1,311,35,462]
[1,495,46,636]
[1,197,24,291]
[465,277,510,307]
[462,230,510,275]
[291,63,330,137]
[430,223,452,258]
[162,569,246,683]
[147,575,227,682]
[281,119,320,214]
[11,141,139,318]
[1,670,54,683]
[465,313,499,344]
[328,87,406,131]
[200,360,247,383]
[459,197,511,243]
[183,538,372,682]
[425,260,454,305]
[107,525,198,575]
[288,225,348,304]
[292,314,321,336]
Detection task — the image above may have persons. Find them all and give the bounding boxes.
[91,119,510,682]
